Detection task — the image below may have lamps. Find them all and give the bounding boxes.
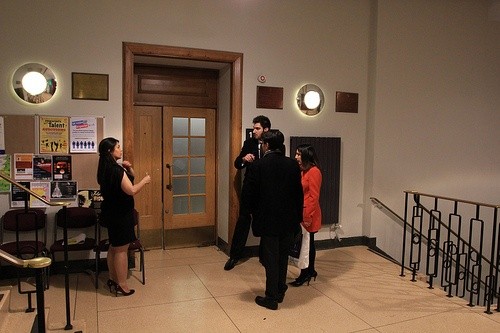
[296,83,325,117]
[12,62,57,105]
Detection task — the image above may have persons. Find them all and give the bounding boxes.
[97,137,152,297]
[243,129,305,310]
[224,116,286,271]
[288,143,322,287]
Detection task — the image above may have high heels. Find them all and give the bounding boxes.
[115,284,135,297]
[288,271,318,287]
[107,279,118,293]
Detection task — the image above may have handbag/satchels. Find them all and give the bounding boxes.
[288,224,310,269]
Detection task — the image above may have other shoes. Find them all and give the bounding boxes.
[254,296,278,310]
[224,257,239,271]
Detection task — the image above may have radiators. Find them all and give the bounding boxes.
[289,136,342,229]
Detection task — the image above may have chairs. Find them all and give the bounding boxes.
[97,207,146,289]
[49,207,97,289]
[0,208,50,290]
